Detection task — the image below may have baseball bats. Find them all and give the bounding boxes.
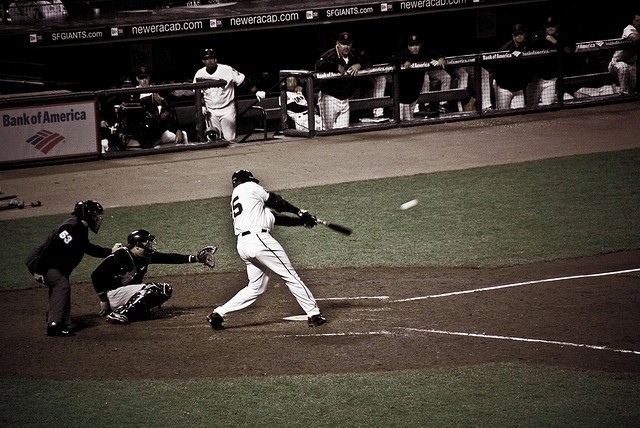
[298,212,353,235]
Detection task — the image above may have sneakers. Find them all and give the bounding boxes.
[206,313,226,331]
[46,322,75,337]
[308,314,326,328]
[106,313,128,325]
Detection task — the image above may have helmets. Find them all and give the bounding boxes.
[127,229,157,261]
[231,168,259,186]
[74,201,103,234]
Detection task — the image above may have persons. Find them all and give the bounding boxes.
[279,77,324,131]
[193,48,266,142]
[494,23,533,110]
[608,14,639,94]
[25,199,125,336]
[531,16,563,105]
[207,169,326,330]
[400,36,446,122]
[91,229,217,323]
[318,33,361,130]
[456,64,493,111]
[99,118,118,154]
[134,64,153,88]
[147,88,184,146]
[421,60,451,113]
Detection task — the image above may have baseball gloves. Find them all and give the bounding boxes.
[196,245,217,268]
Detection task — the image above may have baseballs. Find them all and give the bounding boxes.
[401,200,417,210]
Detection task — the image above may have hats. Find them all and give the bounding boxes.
[136,67,149,78]
[408,34,420,44]
[542,16,558,29]
[337,32,353,43]
[200,47,216,59]
[512,23,524,37]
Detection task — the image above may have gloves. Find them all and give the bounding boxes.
[303,217,316,227]
[299,210,311,219]
